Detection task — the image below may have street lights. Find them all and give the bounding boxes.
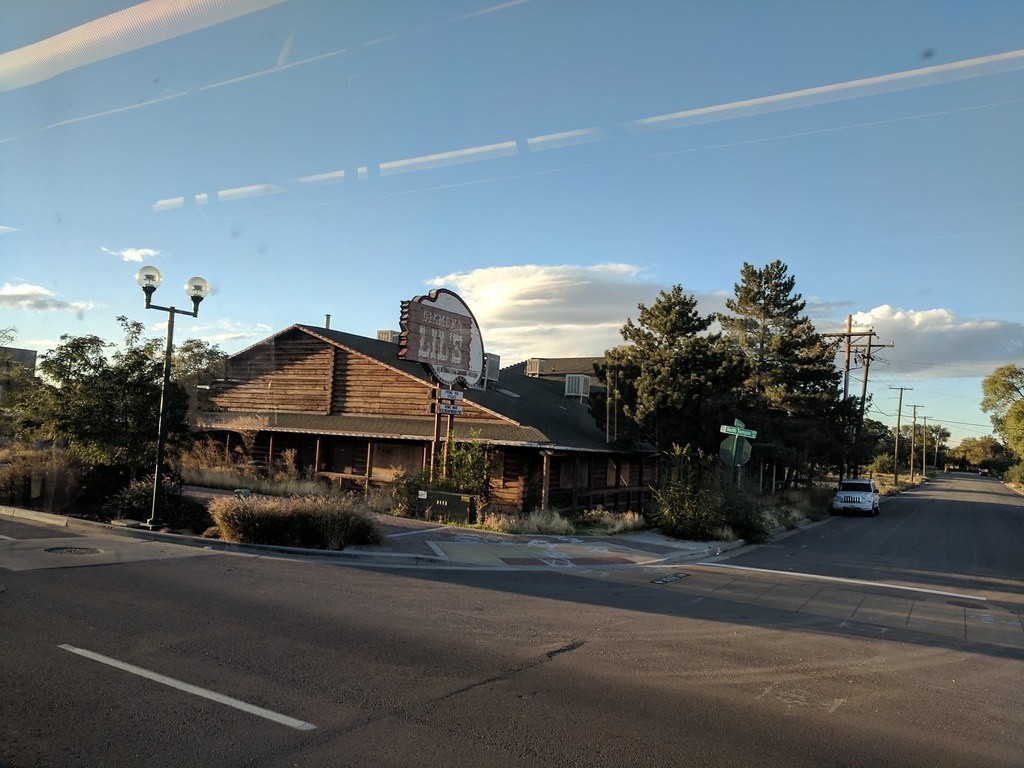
[134,264,210,531]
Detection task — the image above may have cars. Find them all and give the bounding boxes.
[832,479,881,517]
